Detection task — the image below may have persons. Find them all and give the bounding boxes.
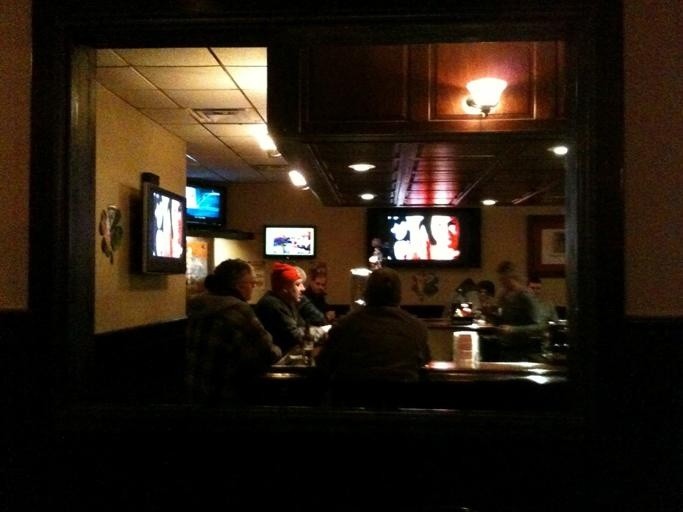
[254,260,327,344]
[482,261,555,363]
[179,258,282,401]
[327,268,432,403]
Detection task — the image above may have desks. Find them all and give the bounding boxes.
[267,307,570,400]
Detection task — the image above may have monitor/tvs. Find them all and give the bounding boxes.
[263,224,317,259]
[186,178,227,229]
[140,181,187,275]
[366,205,482,269]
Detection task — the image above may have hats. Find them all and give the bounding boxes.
[271,262,301,289]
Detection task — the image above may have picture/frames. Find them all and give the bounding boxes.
[525,214,567,277]
